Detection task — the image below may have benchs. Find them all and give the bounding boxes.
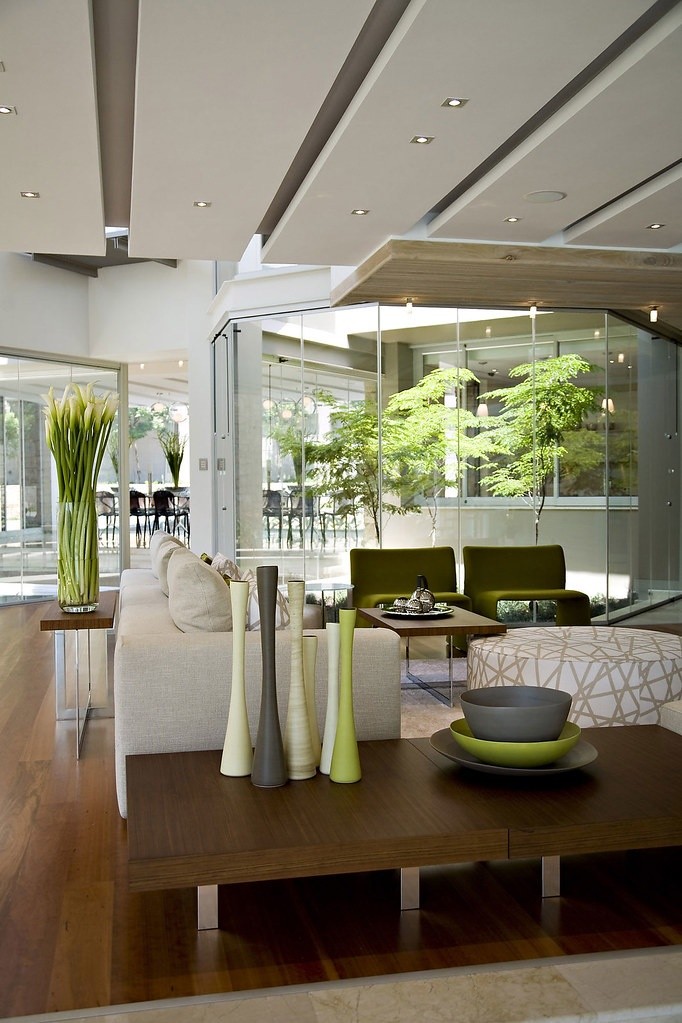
[113,530,401,818]
[350,546,473,652]
[467,627,682,728]
[463,544,591,627]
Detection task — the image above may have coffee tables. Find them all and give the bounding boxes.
[358,606,506,709]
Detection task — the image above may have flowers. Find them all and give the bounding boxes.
[40,379,120,606]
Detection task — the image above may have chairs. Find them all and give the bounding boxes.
[96,491,190,549]
[263,489,358,548]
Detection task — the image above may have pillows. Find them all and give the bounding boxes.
[200,553,291,632]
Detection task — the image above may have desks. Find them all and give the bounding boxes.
[125,723,682,931]
[40,591,118,761]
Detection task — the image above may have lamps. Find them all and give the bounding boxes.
[601,396,614,417]
[477,401,489,418]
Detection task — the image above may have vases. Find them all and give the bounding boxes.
[55,501,100,613]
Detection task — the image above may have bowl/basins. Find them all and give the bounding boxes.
[449,716,581,767]
[460,685,572,743]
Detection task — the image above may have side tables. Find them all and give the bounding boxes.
[280,582,355,629]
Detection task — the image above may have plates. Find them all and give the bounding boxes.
[381,606,454,616]
[429,728,598,777]
